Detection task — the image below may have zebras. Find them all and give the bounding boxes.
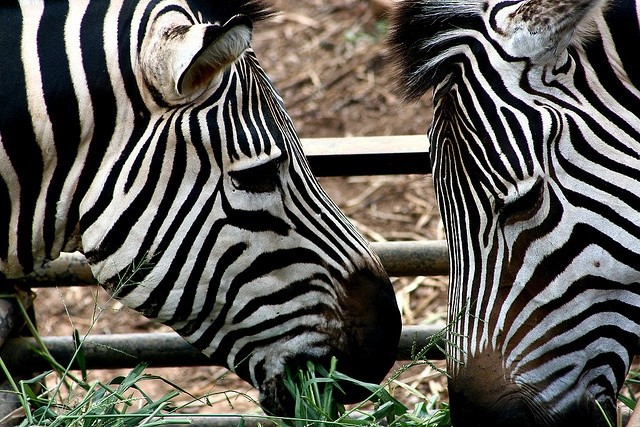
[380,0,640,427]
[1,0,402,427]
